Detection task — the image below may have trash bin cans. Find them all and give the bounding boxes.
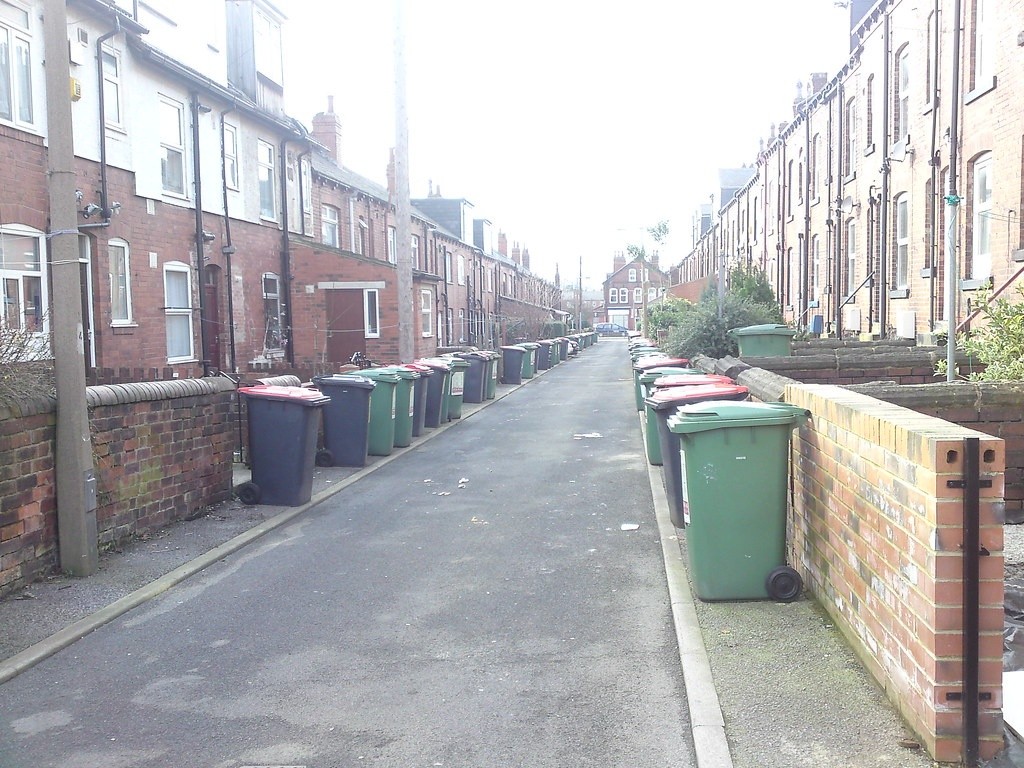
[459,350,502,403]
[342,357,472,456]
[313,373,377,466]
[627,336,751,530]
[514,331,599,379]
[237,385,331,506]
[726,323,797,356]
[667,399,811,602]
[501,346,527,384]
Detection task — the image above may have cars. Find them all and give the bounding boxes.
[595,323,630,337]
